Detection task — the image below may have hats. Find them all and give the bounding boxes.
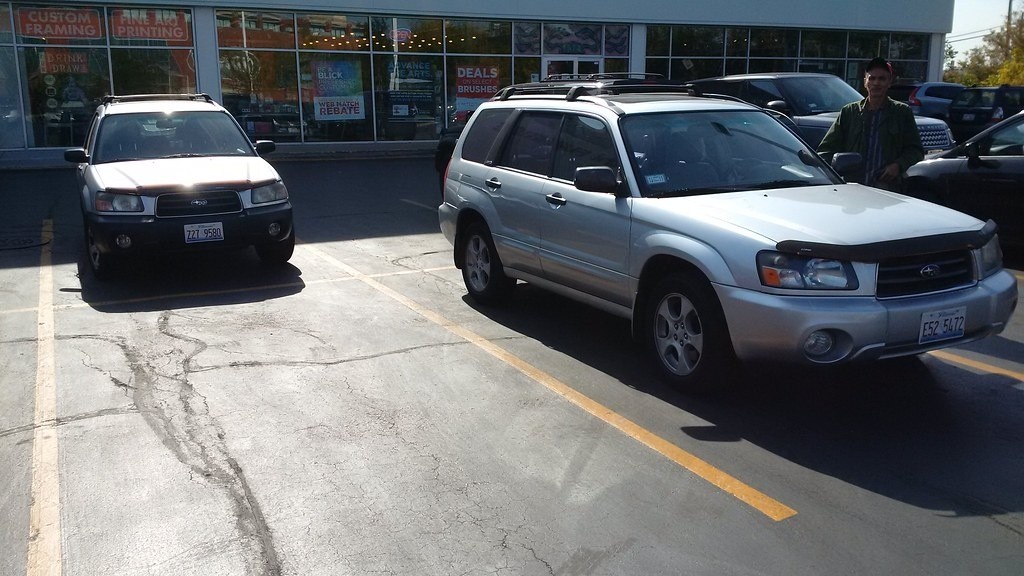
[866,56,893,80]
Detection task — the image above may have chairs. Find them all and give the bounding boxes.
[109,119,158,160]
[657,131,720,189]
[181,116,221,153]
[42,77,93,147]
[590,132,618,179]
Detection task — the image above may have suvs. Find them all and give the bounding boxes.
[908,81,978,119]
[685,71,958,178]
[894,109,1024,255]
[433,72,1018,393]
[62,92,296,279]
[944,84,1024,145]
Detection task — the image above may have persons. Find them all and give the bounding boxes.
[817,57,925,195]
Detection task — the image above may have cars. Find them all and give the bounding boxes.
[317,90,439,141]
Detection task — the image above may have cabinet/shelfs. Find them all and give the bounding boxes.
[241,112,301,142]
